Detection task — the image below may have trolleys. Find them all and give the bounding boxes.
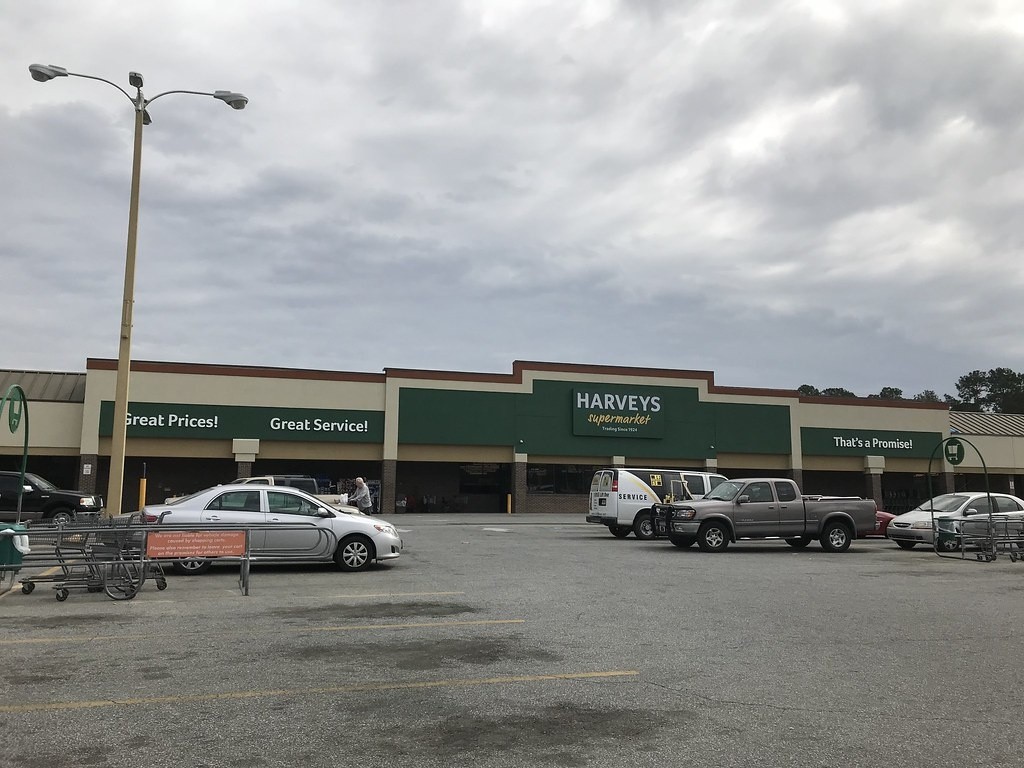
[17,511,172,601]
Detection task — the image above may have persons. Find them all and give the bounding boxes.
[755,485,772,502]
[348,477,373,515]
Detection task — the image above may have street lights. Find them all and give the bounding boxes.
[29,63,249,515]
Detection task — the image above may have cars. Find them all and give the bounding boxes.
[143,484,403,575]
[268,493,365,515]
[857,511,896,538]
[887,492,1024,548]
[0,471,104,526]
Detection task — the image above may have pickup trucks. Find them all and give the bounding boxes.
[165,475,349,512]
[650,478,878,553]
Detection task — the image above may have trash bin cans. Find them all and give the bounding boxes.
[0,522,28,564]
[938,516,956,552]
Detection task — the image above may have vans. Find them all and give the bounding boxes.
[586,468,740,543]
